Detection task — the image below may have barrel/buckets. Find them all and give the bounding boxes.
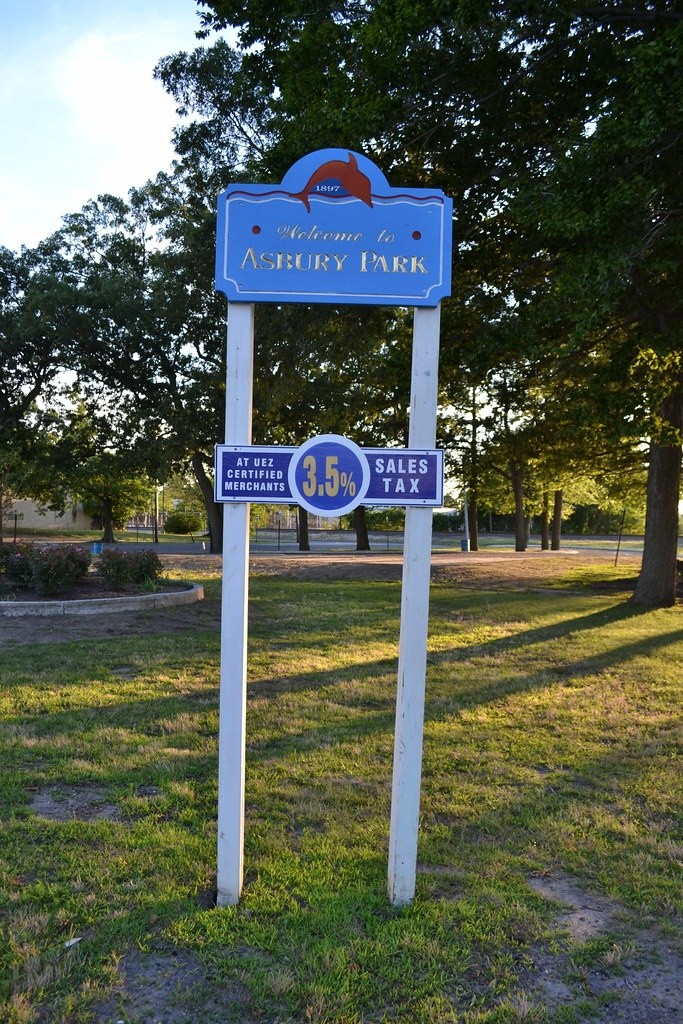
[93,542,103,555]
[460,539,471,551]
